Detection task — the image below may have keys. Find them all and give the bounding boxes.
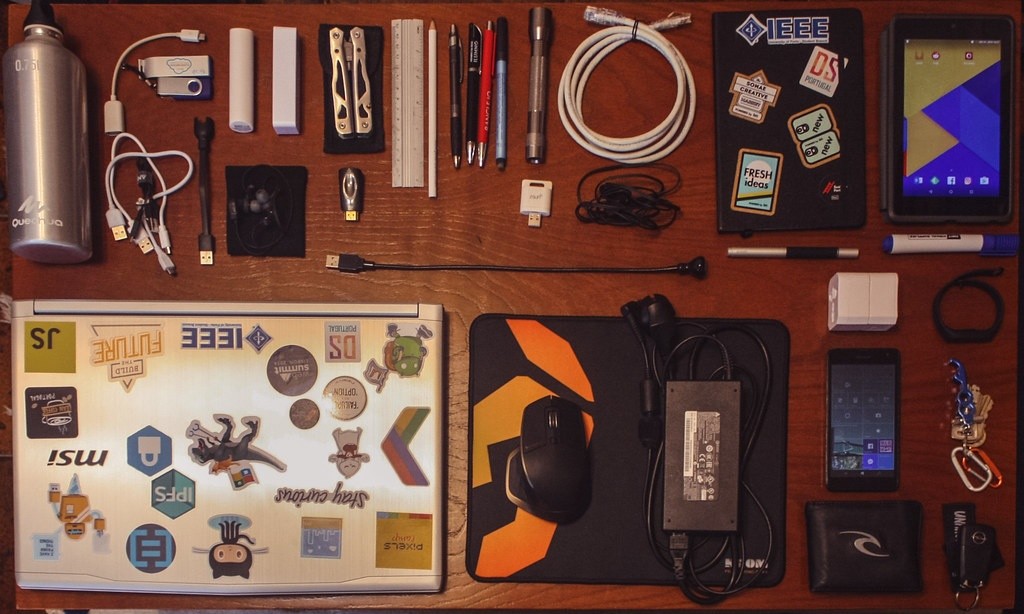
[944,383,997,449]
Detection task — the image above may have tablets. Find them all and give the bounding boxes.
[881,13,1016,224]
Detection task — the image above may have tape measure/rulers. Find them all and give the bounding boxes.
[391,17,424,188]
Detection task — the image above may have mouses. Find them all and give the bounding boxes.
[520,393,593,525]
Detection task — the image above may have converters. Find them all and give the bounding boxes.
[662,377,741,534]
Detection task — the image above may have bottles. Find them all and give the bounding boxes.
[0,1,95,265]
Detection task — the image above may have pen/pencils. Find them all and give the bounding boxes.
[474,18,495,168]
[447,22,463,170]
[728,246,863,263]
[495,14,512,172]
[425,16,439,199]
[466,22,482,167]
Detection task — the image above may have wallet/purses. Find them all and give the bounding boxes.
[805,500,924,594]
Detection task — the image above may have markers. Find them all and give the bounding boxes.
[885,229,1024,260]
[526,6,555,166]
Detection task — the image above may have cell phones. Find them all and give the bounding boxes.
[826,346,900,491]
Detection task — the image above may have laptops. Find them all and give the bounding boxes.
[10,299,444,596]
